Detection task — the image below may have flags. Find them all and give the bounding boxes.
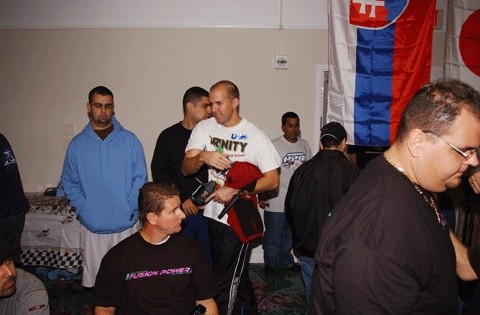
[325,0,480,147]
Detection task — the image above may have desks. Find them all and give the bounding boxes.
[20,196,83,269]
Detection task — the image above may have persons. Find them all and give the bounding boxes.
[0,131,31,269]
[0,247,49,315]
[259,111,314,280]
[61,85,149,288]
[150,79,284,315]
[93,182,218,315]
[308,76,480,315]
[284,121,362,291]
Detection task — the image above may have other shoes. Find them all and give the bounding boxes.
[263,274,294,289]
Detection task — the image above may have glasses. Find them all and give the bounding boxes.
[423,128,480,160]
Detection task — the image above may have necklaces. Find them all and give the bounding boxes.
[384,153,444,226]
